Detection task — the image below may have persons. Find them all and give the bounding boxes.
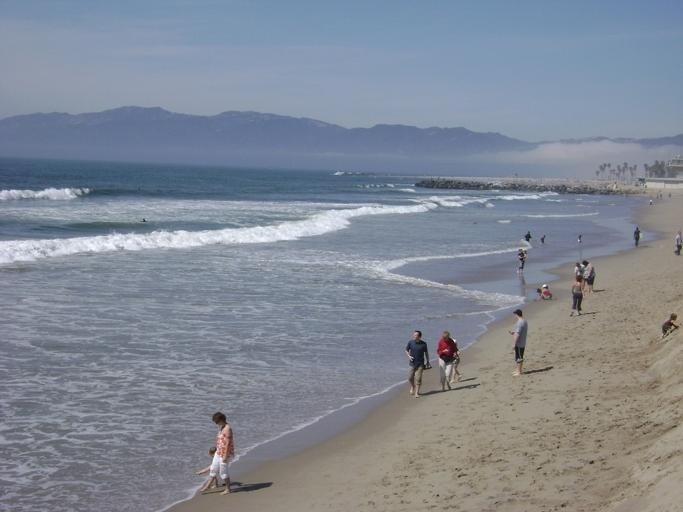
[436,330,458,392]
[633,227,640,247]
[508,308,527,378]
[660,313,680,339]
[673,231,683,256]
[403,330,430,398]
[516,231,596,317]
[451,337,463,385]
[195,447,218,490]
[198,411,235,496]
